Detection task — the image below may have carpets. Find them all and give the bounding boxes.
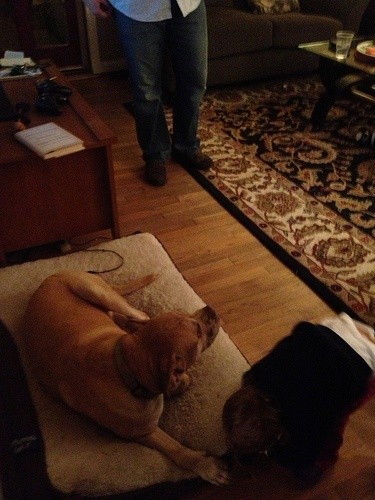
[124,76,375,327]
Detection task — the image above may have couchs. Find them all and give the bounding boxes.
[163,0,365,86]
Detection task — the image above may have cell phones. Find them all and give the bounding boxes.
[40,95,67,102]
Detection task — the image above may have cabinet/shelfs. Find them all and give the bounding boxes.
[0,64,117,254]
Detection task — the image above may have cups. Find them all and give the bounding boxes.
[335,31,354,60]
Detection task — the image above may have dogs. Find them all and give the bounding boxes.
[21,267,239,492]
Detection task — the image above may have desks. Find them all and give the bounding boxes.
[298,36,375,129]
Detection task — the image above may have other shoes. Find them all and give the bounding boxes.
[171,147,214,167]
[147,162,168,187]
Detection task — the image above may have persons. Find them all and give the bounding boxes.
[87,0,215,187]
[221,312,375,488]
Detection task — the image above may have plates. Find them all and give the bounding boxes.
[355,40,375,63]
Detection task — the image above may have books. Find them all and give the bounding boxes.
[14,121,84,160]
